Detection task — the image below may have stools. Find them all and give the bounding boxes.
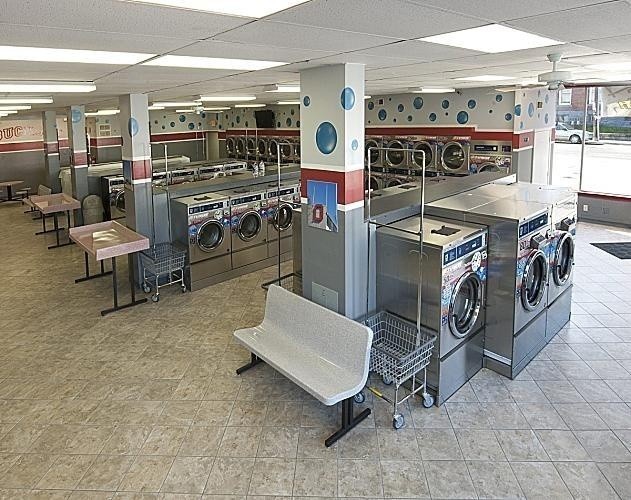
[0,180,31,206]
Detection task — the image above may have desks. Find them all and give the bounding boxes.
[67,219,152,317]
[31,193,81,250]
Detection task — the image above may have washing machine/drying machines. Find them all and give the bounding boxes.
[225,135,470,176]
[57,153,247,225]
[374,179,578,408]
[169,176,302,292]
[471,139,513,174]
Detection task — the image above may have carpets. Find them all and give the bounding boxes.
[589,242,630,260]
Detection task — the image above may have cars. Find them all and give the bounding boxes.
[555,120,594,144]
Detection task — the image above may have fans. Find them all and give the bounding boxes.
[495,52,608,93]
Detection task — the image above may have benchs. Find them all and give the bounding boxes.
[234,283,374,446]
[23,184,54,220]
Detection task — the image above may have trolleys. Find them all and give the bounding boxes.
[259,272,302,305]
[140,242,188,302]
[354,310,437,430]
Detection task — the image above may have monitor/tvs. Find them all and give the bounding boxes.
[255,110,273,128]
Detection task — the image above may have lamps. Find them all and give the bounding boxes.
[0,83,97,92]
[85,108,122,116]
[148,93,266,114]
[411,86,453,94]
[1,96,54,118]
[364,95,372,100]
[264,85,301,106]
